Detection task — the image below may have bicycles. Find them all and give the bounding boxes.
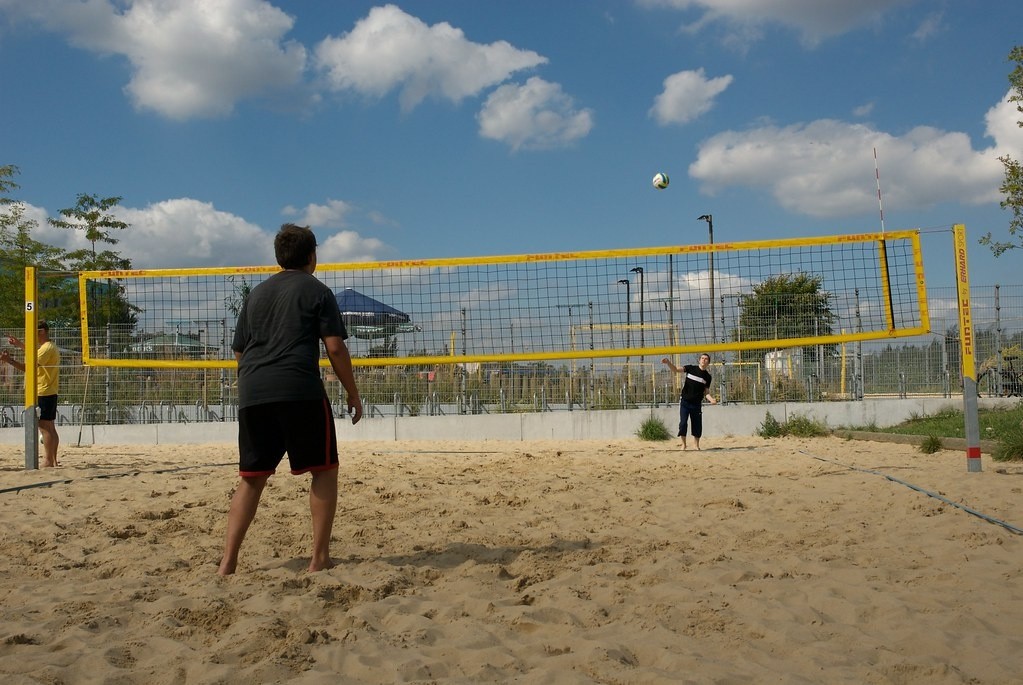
[975,357,1023,399]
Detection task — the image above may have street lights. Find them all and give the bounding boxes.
[697,214,718,363]
[617,279,631,376]
[630,267,647,381]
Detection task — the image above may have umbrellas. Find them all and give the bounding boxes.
[131,333,216,358]
[347,323,421,340]
[327,288,409,358]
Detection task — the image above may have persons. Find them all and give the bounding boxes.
[217,223,363,578]
[662,353,717,451]
[0,321,60,468]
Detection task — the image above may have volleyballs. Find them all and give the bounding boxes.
[653,172,669,189]
